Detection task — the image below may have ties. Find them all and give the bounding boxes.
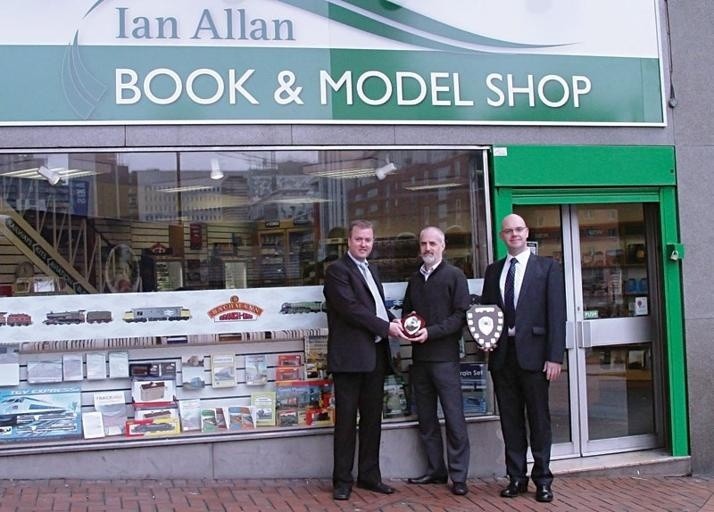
[359,263,391,323]
[503,257,519,329]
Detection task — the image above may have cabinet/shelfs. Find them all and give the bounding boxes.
[525,221,652,382]
[257,225,317,287]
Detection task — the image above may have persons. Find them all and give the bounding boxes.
[401,226,472,496]
[477,213,566,503]
[322,219,408,501]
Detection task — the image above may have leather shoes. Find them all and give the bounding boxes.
[451,481,469,496]
[534,484,556,504]
[356,481,396,494]
[407,473,449,486]
[331,485,353,502]
[500,478,531,499]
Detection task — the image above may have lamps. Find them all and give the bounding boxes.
[209,157,224,181]
[401,175,468,192]
[0,159,112,186]
[302,150,401,180]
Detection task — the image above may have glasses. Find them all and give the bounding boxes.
[501,226,528,233]
